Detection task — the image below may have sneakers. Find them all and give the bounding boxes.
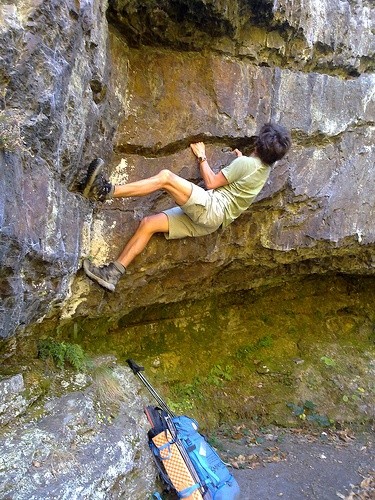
[81,158,115,203]
[82,258,126,291]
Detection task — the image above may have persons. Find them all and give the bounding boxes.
[83,120,292,291]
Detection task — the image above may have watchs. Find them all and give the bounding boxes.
[197,157,205,163]
